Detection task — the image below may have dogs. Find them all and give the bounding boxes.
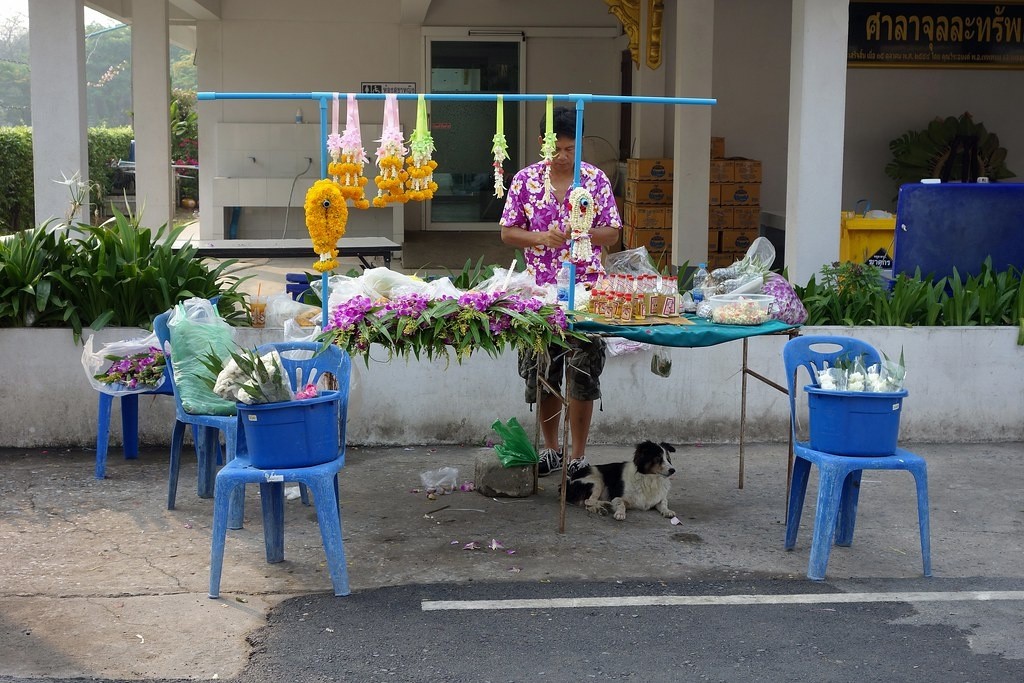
[560,440,678,522]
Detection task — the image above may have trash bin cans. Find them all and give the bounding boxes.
[840,211,896,293]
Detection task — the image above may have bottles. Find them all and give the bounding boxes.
[588,271,678,322]
[295,109,302,124]
[556,262,570,311]
[684,264,717,314]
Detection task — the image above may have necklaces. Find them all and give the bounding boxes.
[326,92,439,211]
[306,179,348,272]
[491,95,556,202]
[567,188,598,278]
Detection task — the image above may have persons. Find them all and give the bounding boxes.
[499,105,623,482]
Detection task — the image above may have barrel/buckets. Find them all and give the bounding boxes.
[805,385,908,456]
[237,390,340,469]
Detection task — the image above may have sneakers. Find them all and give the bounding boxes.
[538,448,563,477]
[567,455,589,480]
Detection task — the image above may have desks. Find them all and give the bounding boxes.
[152,234,402,271]
[318,317,801,525]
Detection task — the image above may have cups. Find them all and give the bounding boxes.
[249,296,267,328]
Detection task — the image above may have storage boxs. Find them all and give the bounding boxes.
[802,385,908,458]
[711,294,775,325]
[623,137,762,276]
[236,391,342,469]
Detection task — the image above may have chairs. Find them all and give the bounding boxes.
[155,304,238,510]
[781,336,931,580]
[208,342,353,596]
[93,365,171,481]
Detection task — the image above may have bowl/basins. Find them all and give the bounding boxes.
[709,294,774,325]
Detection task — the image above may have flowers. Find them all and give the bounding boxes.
[811,350,907,393]
[77,338,166,395]
[202,340,294,407]
[312,264,583,362]
[733,247,808,325]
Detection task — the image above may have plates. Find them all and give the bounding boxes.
[730,277,764,295]
